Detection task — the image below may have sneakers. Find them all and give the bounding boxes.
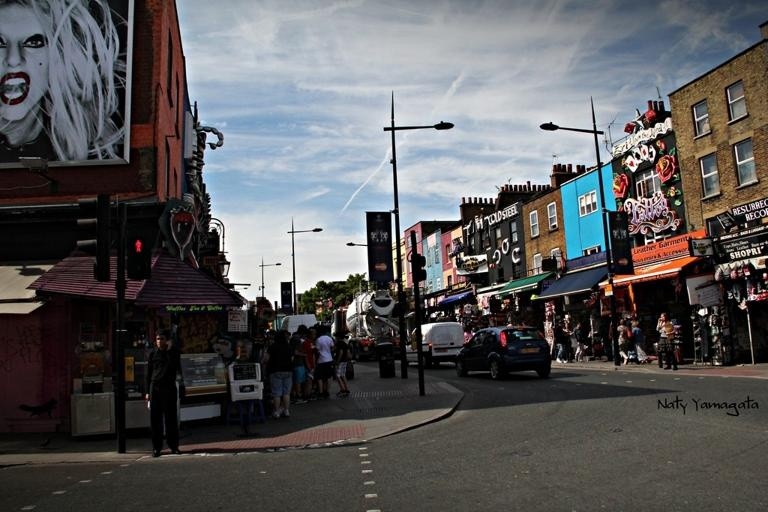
[664,364,678,370]
[274,409,290,418]
[295,389,350,404]
[557,358,582,364]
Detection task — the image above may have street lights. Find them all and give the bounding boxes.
[345,242,368,247]
[258,263,283,297]
[537,121,622,368]
[389,119,457,381]
[286,226,325,315]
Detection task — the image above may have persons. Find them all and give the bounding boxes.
[537,301,652,367]
[264,324,350,421]
[0,0,126,163]
[144,328,182,457]
[656,312,679,371]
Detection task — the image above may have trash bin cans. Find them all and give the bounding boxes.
[377,342,396,378]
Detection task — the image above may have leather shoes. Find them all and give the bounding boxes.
[152,449,180,457]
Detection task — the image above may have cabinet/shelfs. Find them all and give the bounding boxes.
[72,390,264,437]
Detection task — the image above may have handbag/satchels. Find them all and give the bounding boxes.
[627,330,633,337]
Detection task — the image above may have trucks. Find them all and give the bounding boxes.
[280,313,319,335]
[406,321,466,369]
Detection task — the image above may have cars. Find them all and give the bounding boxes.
[455,325,553,380]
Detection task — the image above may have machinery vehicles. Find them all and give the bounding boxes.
[329,287,402,363]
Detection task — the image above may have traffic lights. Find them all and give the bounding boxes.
[75,189,112,284]
[124,212,156,283]
